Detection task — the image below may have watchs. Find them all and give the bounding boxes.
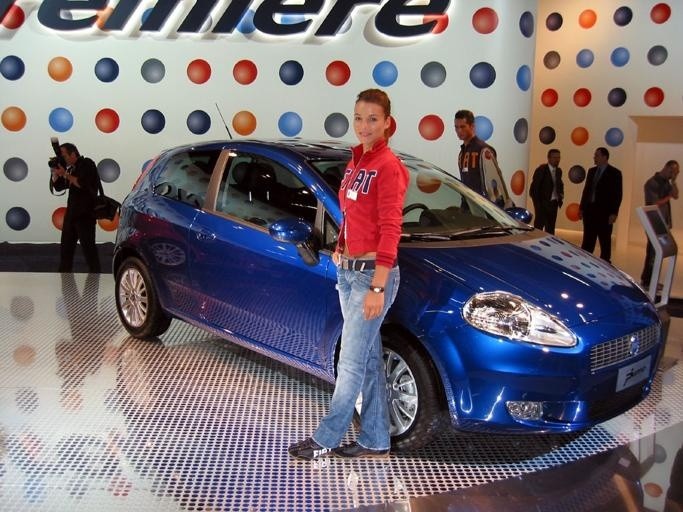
[369,285,384,293]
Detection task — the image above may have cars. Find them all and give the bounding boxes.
[111,100,665,452]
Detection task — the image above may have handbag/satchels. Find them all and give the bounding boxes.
[89,195,122,221]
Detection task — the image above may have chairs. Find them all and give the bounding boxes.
[231,161,278,205]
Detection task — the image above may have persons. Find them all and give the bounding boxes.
[528,149,564,236]
[639,159,679,291]
[578,147,622,264]
[50,143,102,273]
[454,111,514,220]
[287,88,410,460]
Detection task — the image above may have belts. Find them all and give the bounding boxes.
[340,258,398,270]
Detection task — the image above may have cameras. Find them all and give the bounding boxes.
[48,157,66,169]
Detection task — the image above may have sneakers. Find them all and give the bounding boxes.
[333,441,390,459]
[287,437,340,461]
[640,281,665,293]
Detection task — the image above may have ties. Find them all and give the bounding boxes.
[591,167,601,202]
[551,169,559,202]
